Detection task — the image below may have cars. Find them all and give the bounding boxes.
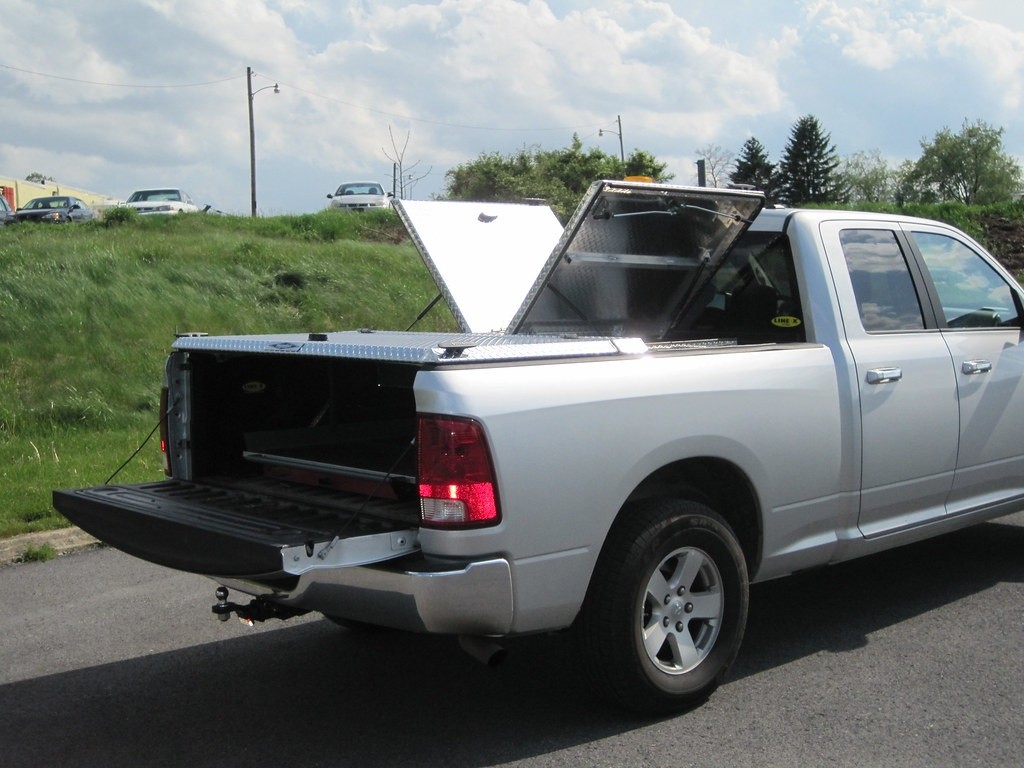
[13,195,99,224]
[0,194,16,225]
[121,188,211,217]
[326,182,395,213]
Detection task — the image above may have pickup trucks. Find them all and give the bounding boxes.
[47,179,1024,715]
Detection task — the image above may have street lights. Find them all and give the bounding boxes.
[246,83,282,218]
[393,173,413,199]
[599,128,626,165]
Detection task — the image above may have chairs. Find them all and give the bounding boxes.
[347,190,354,195]
[369,187,378,194]
[42,203,50,208]
